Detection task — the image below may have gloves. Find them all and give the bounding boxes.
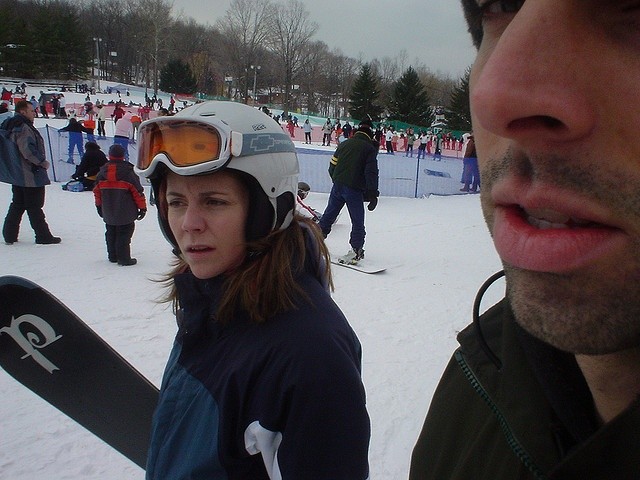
[96,205,103,218]
[137,209,147,220]
[136,208,147,220]
[368,194,377,211]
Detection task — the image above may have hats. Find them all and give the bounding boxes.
[109,145,126,160]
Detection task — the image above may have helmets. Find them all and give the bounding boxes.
[298,182,310,193]
[132,101,300,246]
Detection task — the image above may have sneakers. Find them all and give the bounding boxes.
[36,237,61,244]
[118,258,137,265]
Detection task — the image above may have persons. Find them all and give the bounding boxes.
[29,96,39,118]
[38,91,49,119]
[169,96,175,114]
[385,125,396,155]
[58,118,94,164]
[58,93,67,119]
[445,132,451,150]
[273,114,281,126]
[295,181,322,223]
[392,131,399,152]
[441,132,447,149]
[86,94,90,100]
[143,102,150,120]
[342,120,352,140]
[408,0,638,479]
[135,98,370,480]
[418,130,430,160]
[16,85,21,92]
[116,90,121,97]
[452,135,457,151]
[460,127,480,192]
[127,91,130,96]
[52,95,60,119]
[113,112,134,163]
[137,103,145,121]
[110,102,125,126]
[90,87,113,94]
[332,118,342,149]
[400,133,409,154]
[459,135,464,151]
[21,83,27,93]
[301,119,314,144]
[375,122,384,142]
[0,100,62,244]
[321,117,333,146]
[317,118,380,265]
[131,116,142,141]
[433,134,443,161]
[92,144,148,266]
[96,105,106,140]
[406,129,416,158]
[379,125,388,150]
[62,86,66,91]
[67,82,88,93]
[427,131,434,155]
[69,141,109,191]
[157,108,168,117]
[285,120,295,138]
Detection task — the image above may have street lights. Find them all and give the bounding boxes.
[251,65,261,97]
[93,37,102,93]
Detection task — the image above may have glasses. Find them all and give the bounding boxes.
[133,116,297,178]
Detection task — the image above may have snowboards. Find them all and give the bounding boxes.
[423,168,451,178]
[330,252,387,274]
[0,275,159,472]
